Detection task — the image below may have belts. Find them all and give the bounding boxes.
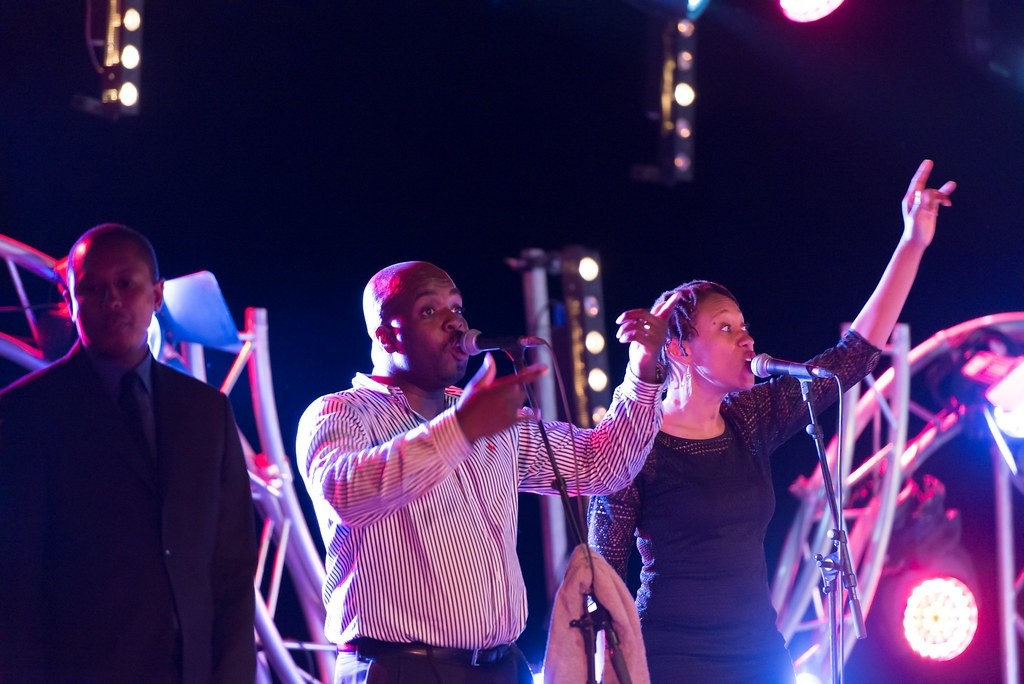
[338,632,513,667]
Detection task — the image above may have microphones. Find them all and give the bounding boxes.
[458,329,547,357]
[751,353,834,379]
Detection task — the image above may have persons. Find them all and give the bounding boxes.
[1,226,261,684]
[296,260,670,684]
[588,160,958,684]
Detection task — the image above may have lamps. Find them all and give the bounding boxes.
[564,249,612,428]
[101,0,142,115]
[660,19,695,180]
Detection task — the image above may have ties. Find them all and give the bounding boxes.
[119,370,156,482]
